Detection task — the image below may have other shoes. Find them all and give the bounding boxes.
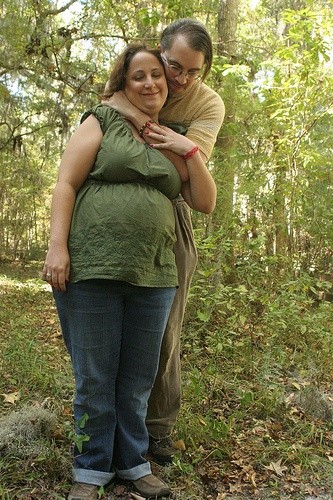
[131,473,170,497]
[145,434,184,467]
[67,481,100,500]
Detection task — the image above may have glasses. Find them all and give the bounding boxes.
[161,57,201,81]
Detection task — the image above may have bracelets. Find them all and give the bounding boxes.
[184,146,199,161]
[139,120,159,139]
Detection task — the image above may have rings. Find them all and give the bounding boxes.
[46,273,51,277]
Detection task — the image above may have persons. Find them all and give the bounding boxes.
[103,18,225,464]
[40,45,218,500]
